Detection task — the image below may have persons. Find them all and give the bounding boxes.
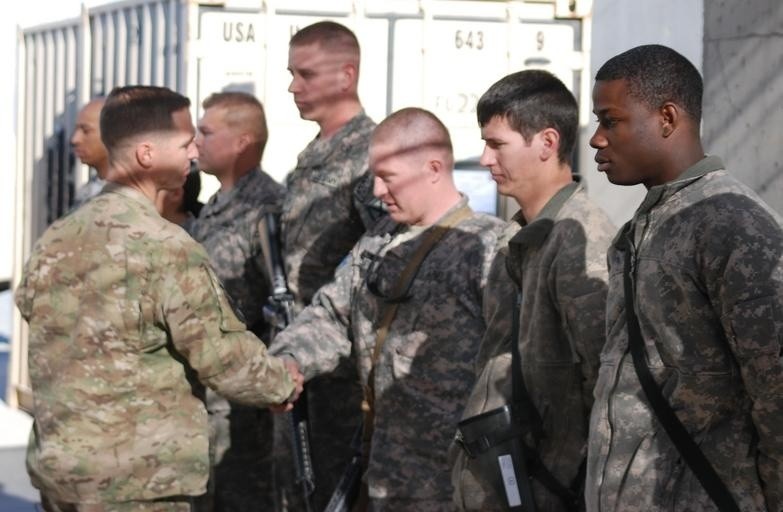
[15,84,307,511]
[151,157,216,511]
[475,69,621,512]
[262,105,511,511]
[583,41,783,510]
[68,95,110,209]
[187,89,289,512]
[279,20,391,512]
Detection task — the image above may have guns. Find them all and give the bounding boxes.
[262,211,320,512]
[324,421,364,512]
[454,407,537,512]
